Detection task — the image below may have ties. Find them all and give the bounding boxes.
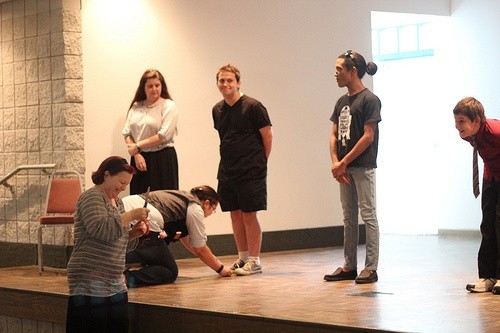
[470,139,480,197]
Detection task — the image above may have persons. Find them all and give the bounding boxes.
[212,65,272,275]
[324,50,382,284]
[122,186,234,289]
[66,156,151,333]
[453,97,500,294]
[121,68,178,195]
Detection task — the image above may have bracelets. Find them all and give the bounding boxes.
[216,265,224,273]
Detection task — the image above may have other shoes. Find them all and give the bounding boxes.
[323,266,378,284]
[465,277,499,295]
[224,257,263,275]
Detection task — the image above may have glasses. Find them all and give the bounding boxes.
[209,204,216,212]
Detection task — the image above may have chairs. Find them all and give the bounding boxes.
[37,169,84,276]
